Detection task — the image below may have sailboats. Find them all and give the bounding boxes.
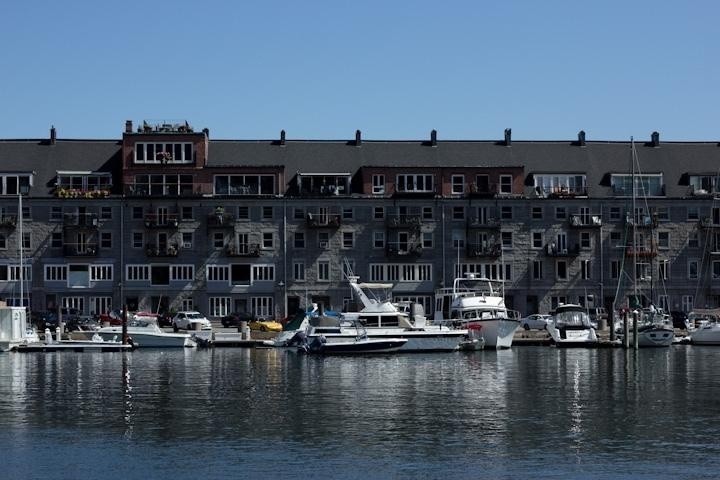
[612,136,720,348]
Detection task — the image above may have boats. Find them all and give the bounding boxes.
[0,194,197,350]
[302,256,521,355]
[545,301,597,348]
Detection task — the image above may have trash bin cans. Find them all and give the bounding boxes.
[598,319,607,330]
[192,323,201,331]
[104,321,110,327]
[689,318,695,327]
[238,321,247,332]
[242,326,250,339]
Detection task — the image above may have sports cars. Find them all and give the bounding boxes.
[221,312,283,332]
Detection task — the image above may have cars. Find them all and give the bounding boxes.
[521,313,551,330]
[171,310,212,331]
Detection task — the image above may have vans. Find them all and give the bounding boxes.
[590,307,608,320]
